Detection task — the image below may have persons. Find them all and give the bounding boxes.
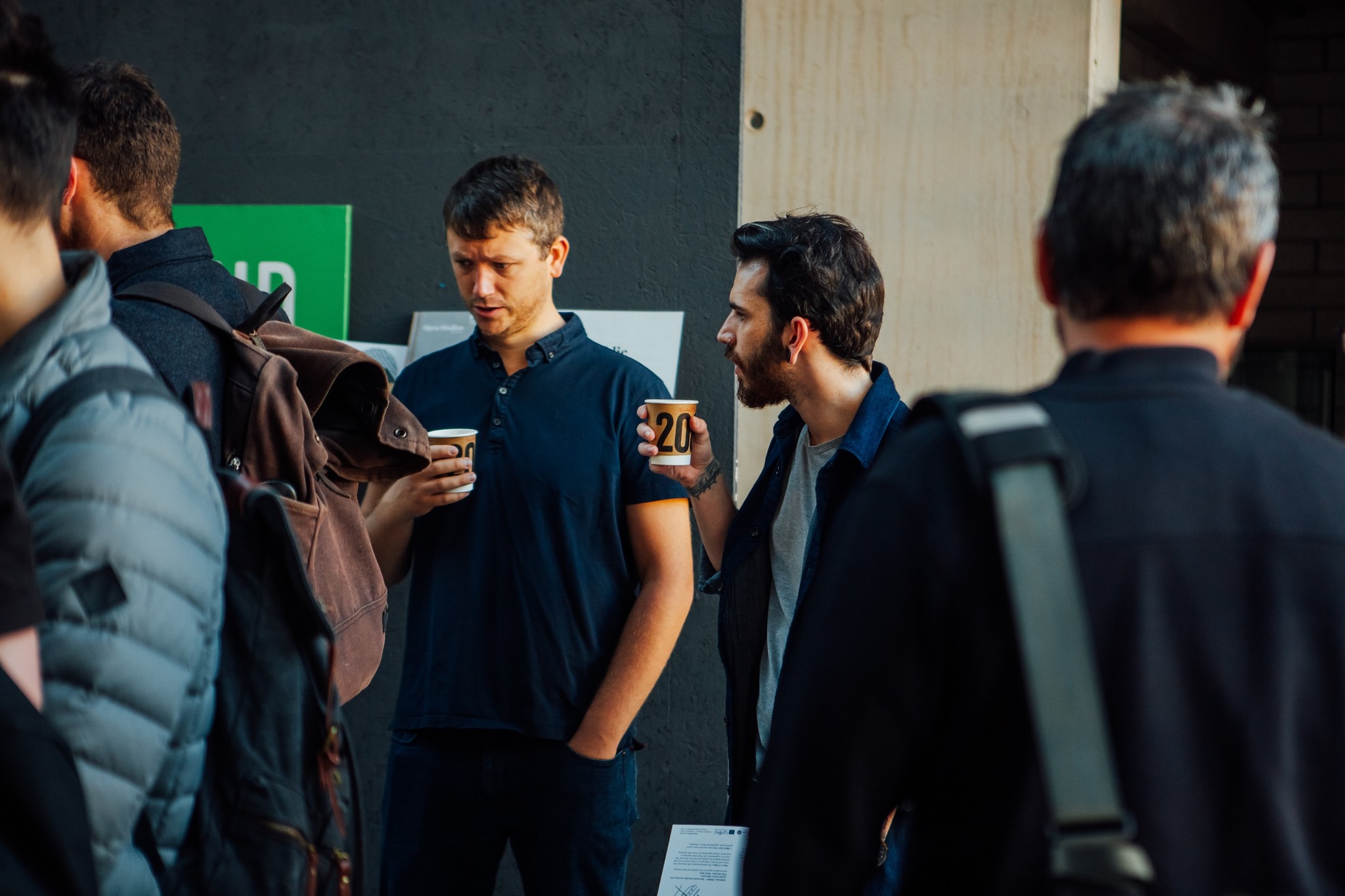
[637,213,915,896]
[0,50,362,895]
[363,154,699,896]
[723,73,1345,896]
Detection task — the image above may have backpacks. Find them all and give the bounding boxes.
[114,277,432,709]
[11,363,362,896]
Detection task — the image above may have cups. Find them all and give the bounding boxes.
[644,399,699,465]
[428,429,478,493]
